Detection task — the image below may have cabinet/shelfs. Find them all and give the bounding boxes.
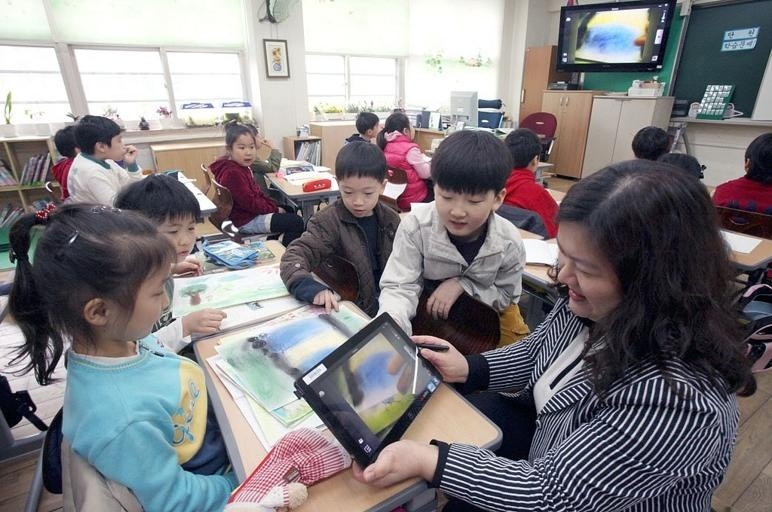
[520,45,571,147]
[539,89,602,181]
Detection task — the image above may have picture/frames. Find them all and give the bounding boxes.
[264,38,291,78]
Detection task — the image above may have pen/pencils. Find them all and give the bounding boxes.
[416,344,449,350]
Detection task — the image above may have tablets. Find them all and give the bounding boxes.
[293,312,444,470]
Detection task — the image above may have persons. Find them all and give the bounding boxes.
[631,127,704,178]
[0,111,239,512]
[710,131,771,215]
[207,122,304,249]
[279,111,532,353]
[346,160,747,512]
[496,125,559,238]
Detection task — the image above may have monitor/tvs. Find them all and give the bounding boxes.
[556,0,677,73]
[450,90,478,131]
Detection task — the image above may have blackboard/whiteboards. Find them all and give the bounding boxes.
[667,0,772,127]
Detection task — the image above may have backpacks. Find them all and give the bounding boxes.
[729,283,772,372]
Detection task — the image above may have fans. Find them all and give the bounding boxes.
[255,0,302,23]
